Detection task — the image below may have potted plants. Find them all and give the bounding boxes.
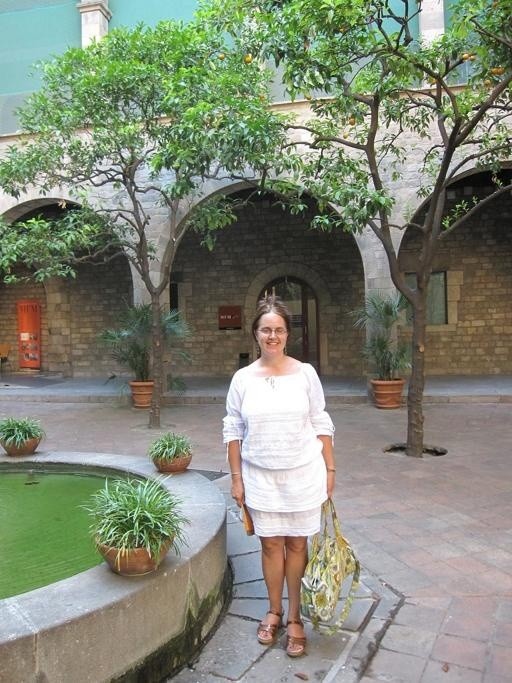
[94,291,191,408]
[76,429,200,579]
[342,292,413,410]
[0,412,49,458]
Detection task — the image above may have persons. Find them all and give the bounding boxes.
[221,295,337,657]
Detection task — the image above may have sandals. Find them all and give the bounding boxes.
[256,608,285,644]
[286,618,306,657]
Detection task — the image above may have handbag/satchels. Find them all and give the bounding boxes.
[299,496,360,637]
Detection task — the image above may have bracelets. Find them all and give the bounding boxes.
[327,468,337,472]
[231,472,242,475]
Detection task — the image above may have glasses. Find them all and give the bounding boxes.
[256,328,287,336]
